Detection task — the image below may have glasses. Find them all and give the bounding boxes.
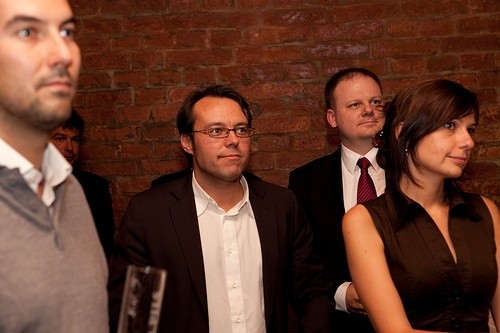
[194,127,255,138]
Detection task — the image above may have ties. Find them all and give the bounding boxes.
[356,157,378,206]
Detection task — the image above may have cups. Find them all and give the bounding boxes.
[116,265,166,333]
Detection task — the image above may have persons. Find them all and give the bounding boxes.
[0,0,116,333]
[288,68,386,333]
[108,84,338,333]
[342,78,500,333]
